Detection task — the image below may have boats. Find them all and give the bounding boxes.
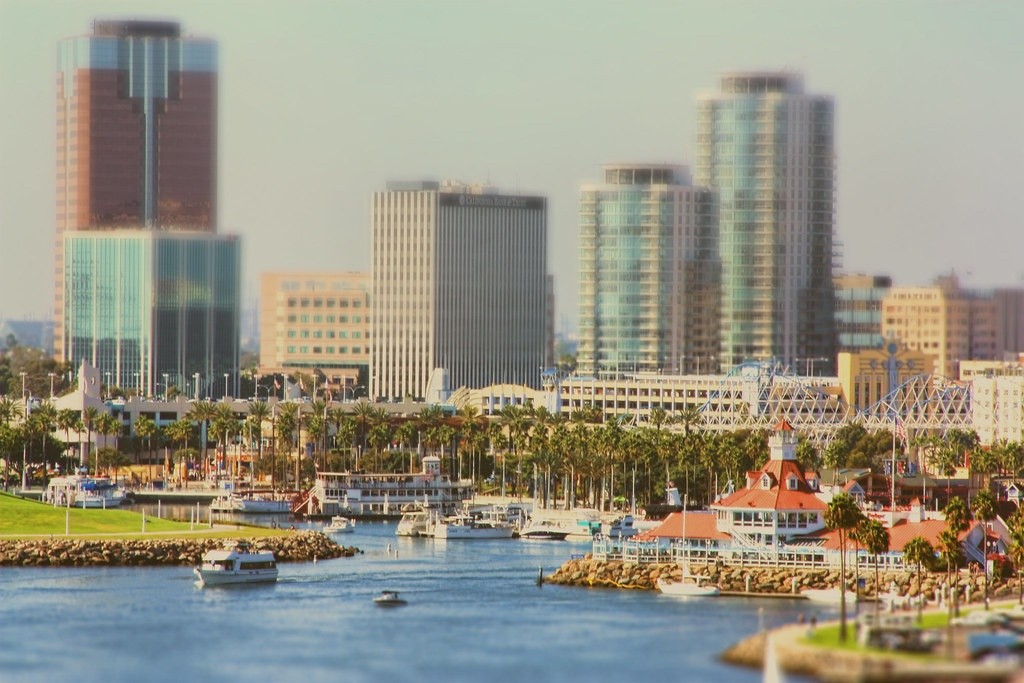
[193,538,280,584]
[373,588,409,607]
[208,492,293,514]
[800,585,858,606]
[46,471,123,508]
[655,574,722,598]
[396,496,575,541]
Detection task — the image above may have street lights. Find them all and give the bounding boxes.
[105,372,112,398]
[162,374,170,402]
[311,374,320,403]
[254,374,259,403]
[224,374,230,398]
[48,373,57,402]
[19,372,28,402]
[134,373,140,396]
[342,385,365,401]
